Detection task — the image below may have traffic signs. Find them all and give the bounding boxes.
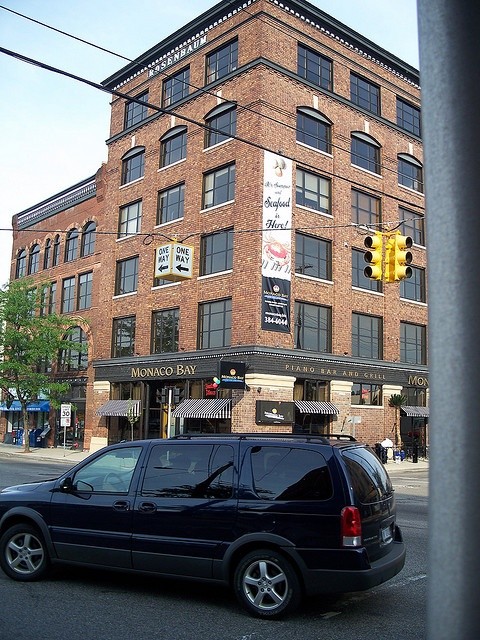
[154,241,193,280]
[61,404,71,427]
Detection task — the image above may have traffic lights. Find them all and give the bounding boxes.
[363,230,382,282]
[392,231,413,282]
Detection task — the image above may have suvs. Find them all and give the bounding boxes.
[0,433,407,620]
